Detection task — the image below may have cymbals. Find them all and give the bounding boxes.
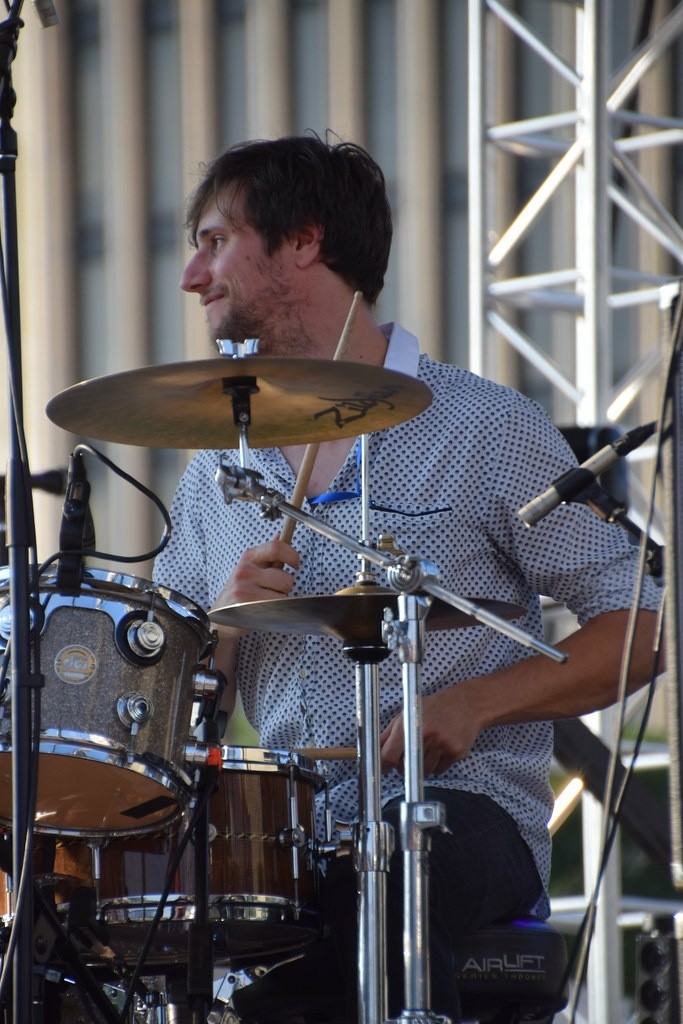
[44,354,435,451]
[205,582,530,646]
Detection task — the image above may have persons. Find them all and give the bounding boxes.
[154,128,672,1024]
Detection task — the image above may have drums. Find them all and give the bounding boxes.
[1,747,325,966]
[0,565,224,841]
